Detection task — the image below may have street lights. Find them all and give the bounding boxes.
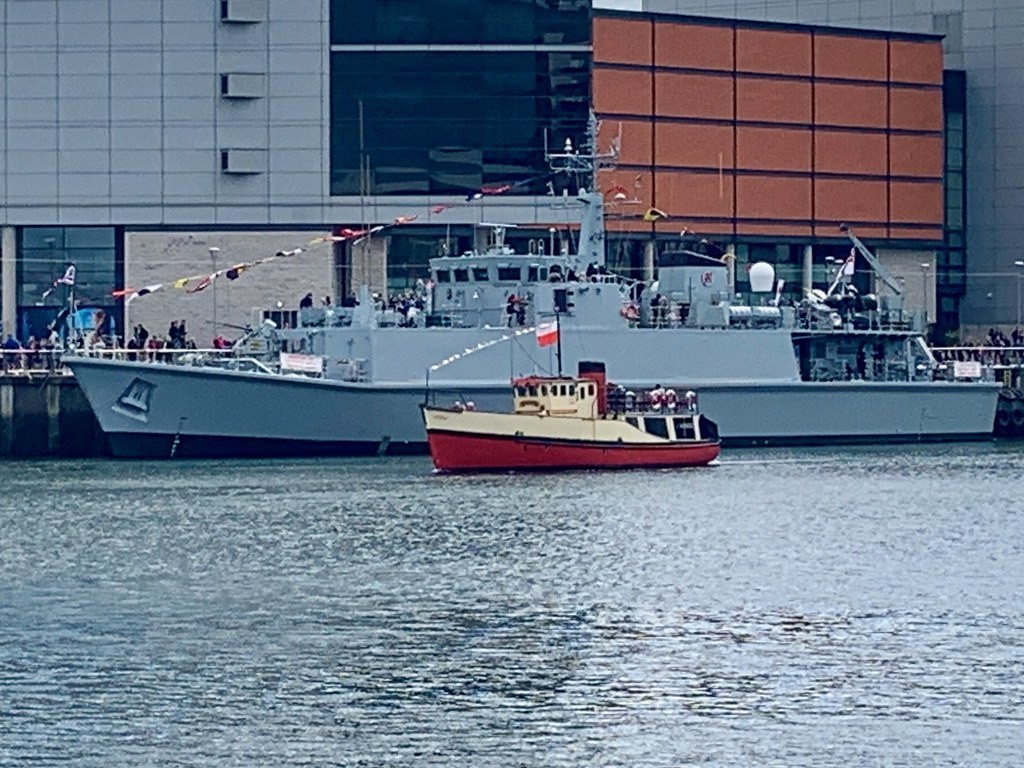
[1013,260,1024,340]
[207,247,221,349]
[920,263,930,348]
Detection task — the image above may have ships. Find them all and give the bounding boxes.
[54,115,1010,469]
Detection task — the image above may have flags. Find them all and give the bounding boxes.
[821,245,856,292]
[94,188,509,295]
[586,169,729,257]
[534,322,557,348]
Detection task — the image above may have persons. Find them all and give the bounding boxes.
[502,296,526,324]
[633,272,1024,403]
[1,278,433,363]
[596,375,672,416]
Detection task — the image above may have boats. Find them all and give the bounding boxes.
[415,307,724,472]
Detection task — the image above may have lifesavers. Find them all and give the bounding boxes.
[624,390,637,410]
[665,388,679,410]
[684,390,697,411]
[626,304,638,316]
[701,271,714,288]
[648,389,662,412]
